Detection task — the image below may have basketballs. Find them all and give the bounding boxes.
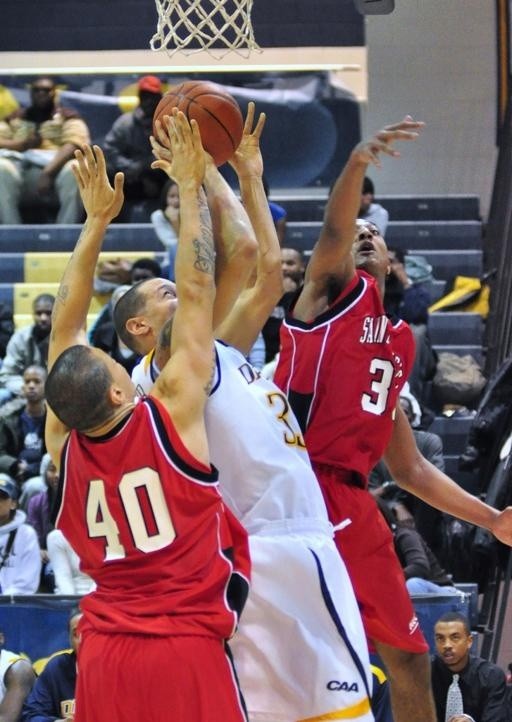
[153,80,243,168]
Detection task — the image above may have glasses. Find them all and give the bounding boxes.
[31,87,53,93]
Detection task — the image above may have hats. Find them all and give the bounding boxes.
[139,76,162,94]
[0,472,18,500]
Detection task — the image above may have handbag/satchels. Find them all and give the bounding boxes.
[441,275,492,322]
[434,352,488,394]
[405,252,432,283]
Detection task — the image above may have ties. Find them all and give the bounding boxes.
[445,675,463,722]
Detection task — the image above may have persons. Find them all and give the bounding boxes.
[3,69,512,722]
